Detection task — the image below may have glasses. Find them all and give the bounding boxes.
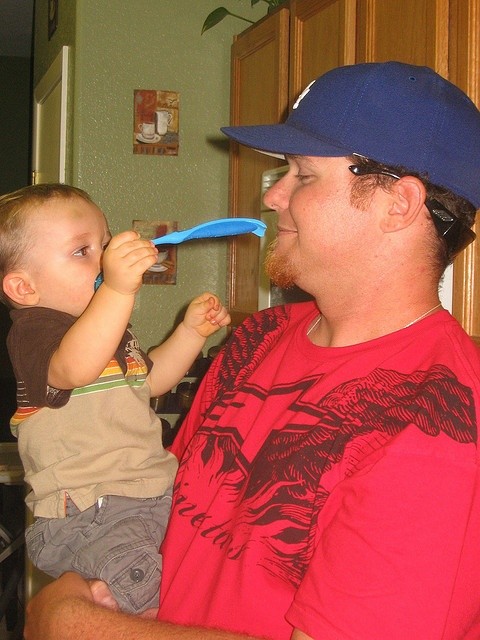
[349,164,477,262]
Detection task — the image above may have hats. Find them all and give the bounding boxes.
[220,61,480,210]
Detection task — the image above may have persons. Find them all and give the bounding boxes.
[1,182,232,614]
[23,61,479,639]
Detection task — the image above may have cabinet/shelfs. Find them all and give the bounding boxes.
[356,2,479,345]
[225,1,357,330]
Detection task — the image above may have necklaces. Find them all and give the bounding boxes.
[306,302,442,338]
